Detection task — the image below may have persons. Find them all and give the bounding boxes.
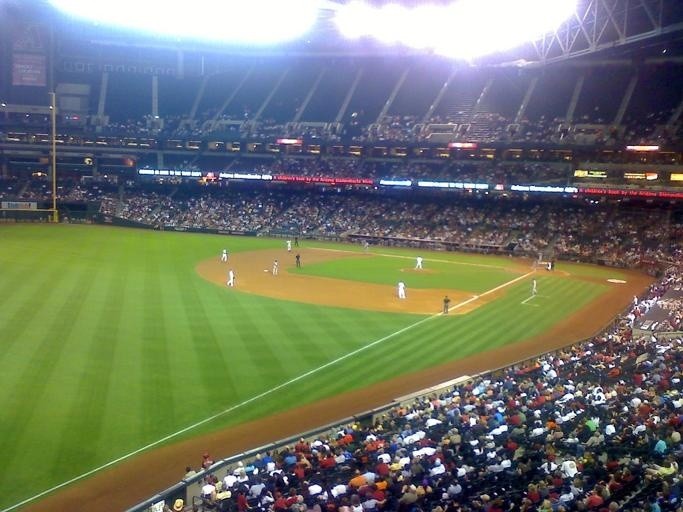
[444,296,450,314]
[398,281,406,298]
[416,256,424,270]
[273,259,278,276]
[227,269,234,287]
[2,103,682,268]
[567,266,682,357]
[532,279,536,294]
[147,353,682,511]
